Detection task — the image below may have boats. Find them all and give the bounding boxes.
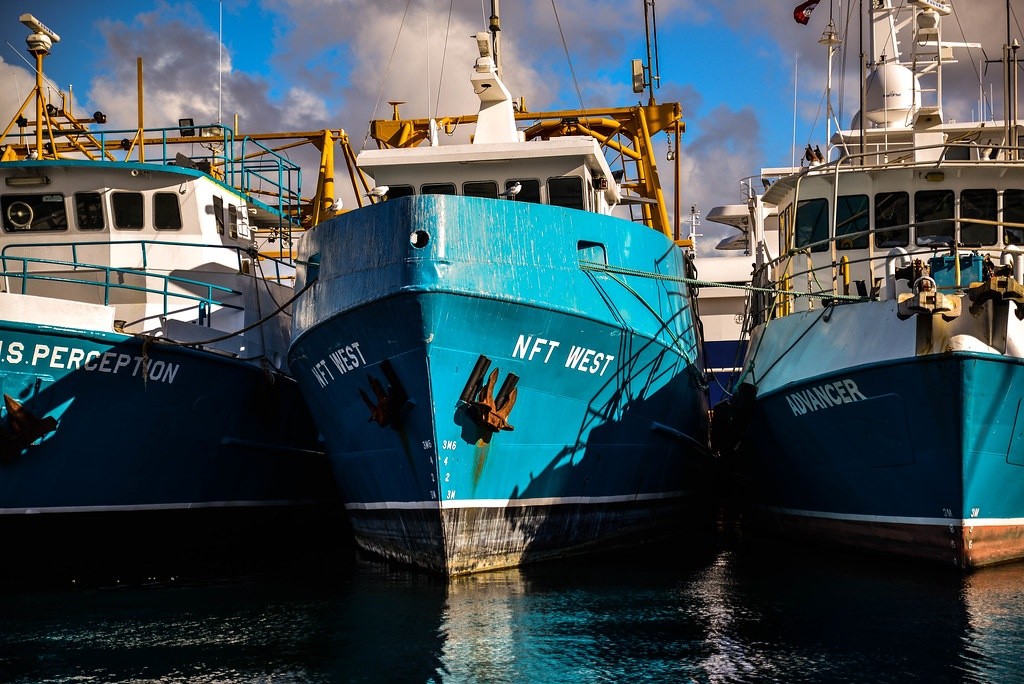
[731,0,1023,573]
[287,0,716,578]
[681,173,780,410]
[0,0,350,581]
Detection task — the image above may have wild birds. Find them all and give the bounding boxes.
[361,185,389,203]
[497,182,523,202]
[324,197,344,219]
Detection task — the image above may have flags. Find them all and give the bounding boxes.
[794,0,821,25]
